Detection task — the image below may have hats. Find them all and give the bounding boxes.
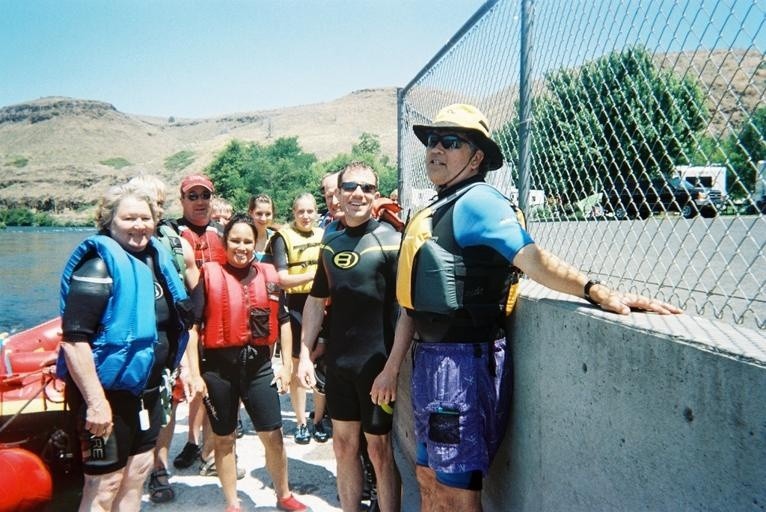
[412,102,505,172]
[179,174,215,195]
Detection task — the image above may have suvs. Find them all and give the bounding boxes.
[603,174,724,221]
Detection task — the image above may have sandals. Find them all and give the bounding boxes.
[146,468,175,503]
[235,419,244,439]
[199,451,247,481]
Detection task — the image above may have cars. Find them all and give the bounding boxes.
[563,192,603,219]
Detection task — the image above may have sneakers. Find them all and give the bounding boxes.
[294,423,311,445]
[311,422,329,443]
[276,493,314,512]
[173,442,202,469]
[308,407,332,439]
[224,501,244,512]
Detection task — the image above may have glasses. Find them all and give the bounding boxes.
[425,131,480,151]
[182,190,212,201]
[336,181,378,194]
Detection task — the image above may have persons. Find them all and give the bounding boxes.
[295,161,405,512]
[185,213,308,512]
[396,103,683,512]
[87,171,329,501]
[57,189,189,512]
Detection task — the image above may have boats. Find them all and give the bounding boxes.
[0,316,70,512]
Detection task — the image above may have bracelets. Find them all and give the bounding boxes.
[584,279,600,305]
